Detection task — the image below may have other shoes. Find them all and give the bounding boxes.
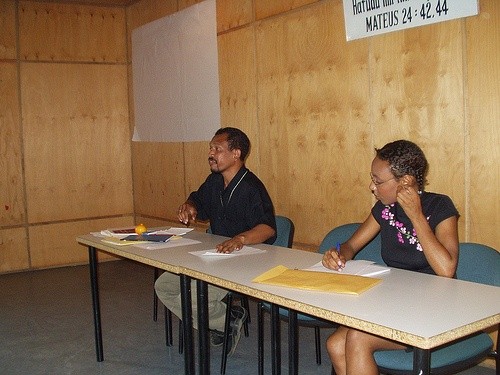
[226,305,249,358]
[210,332,225,347]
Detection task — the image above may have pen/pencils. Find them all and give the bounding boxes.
[336,241,342,272]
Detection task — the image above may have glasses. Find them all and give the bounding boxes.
[369,171,398,187]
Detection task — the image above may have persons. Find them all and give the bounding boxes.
[323,140,460,375]
[155,127,278,358]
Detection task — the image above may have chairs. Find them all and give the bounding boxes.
[257,220,386,375]
[178,216,294,374]
[331,243,500,375]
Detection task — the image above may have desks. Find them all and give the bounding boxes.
[78,227,500,375]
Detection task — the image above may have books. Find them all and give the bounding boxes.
[113,229,174,243]
